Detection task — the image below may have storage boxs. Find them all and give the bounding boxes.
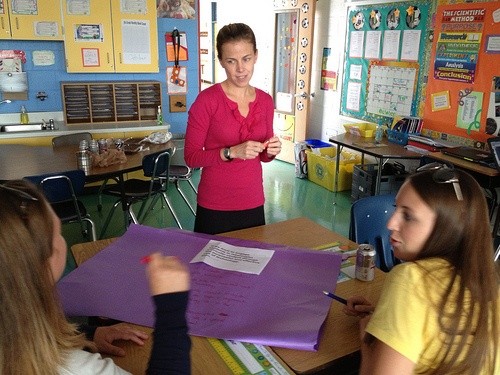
[343,122,378,138]
[304,138,413,205]
[386,128,420,146]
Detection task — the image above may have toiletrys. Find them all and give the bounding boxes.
[374,120,384,142]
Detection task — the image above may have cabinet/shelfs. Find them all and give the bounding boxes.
[60,0,160,73]
[0,0,63,40]
[61,79,162,126]
[84,129,169,195]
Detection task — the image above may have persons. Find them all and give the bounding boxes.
[183,21,282,236]
[344,161,500,375]
[0,179,191,375]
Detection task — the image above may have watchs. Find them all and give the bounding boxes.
[223,145,234,161]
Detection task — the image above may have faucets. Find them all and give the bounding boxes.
[0,100,12,104]
[42,118,55,130]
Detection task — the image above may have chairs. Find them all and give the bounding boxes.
[23,132,199,243]
[350,193,408,273]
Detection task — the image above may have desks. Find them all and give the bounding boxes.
[330,132,423,204]
[426,151,500,238]
[0,137,174,182]
[71,216,389,375]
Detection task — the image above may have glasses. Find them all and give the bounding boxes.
[417,161,468,214]
[0,183,38,227]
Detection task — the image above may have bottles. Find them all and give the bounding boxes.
[20,106,29,124]
[79,137,124,157]
[156,106,163,126]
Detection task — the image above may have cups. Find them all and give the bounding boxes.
[77,150,92,171]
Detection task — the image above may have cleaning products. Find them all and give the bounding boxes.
[20,105,30,124]
[157,105,163,125]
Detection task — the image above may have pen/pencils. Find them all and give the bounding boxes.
[321,289,347,305]
[141,257,151,264]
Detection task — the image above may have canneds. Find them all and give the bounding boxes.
[79,137,124,155]
[355,243,377,282]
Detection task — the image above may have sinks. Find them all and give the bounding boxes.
[0,124,42,132]
